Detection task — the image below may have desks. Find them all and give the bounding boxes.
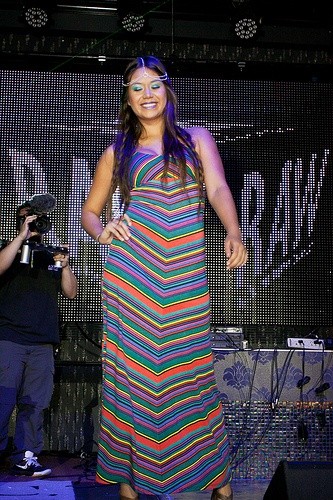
[212,348,333,480]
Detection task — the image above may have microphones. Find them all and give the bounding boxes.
[30,193,57,212]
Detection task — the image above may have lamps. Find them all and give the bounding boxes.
[117,0,147,32]
[23,0,50,28]
[228,0,260,40]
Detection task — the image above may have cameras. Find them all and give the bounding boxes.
[19,209,52,242]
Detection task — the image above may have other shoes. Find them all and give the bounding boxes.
[210,489,233,500]
[118,490,139,500]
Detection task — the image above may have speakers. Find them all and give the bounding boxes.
[262,459,333,500]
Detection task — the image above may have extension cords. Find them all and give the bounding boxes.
[287,338,325,350]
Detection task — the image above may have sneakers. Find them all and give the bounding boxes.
[10,450,52,476]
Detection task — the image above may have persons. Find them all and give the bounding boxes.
[0,193,78,478]
[81,55,249,499]
